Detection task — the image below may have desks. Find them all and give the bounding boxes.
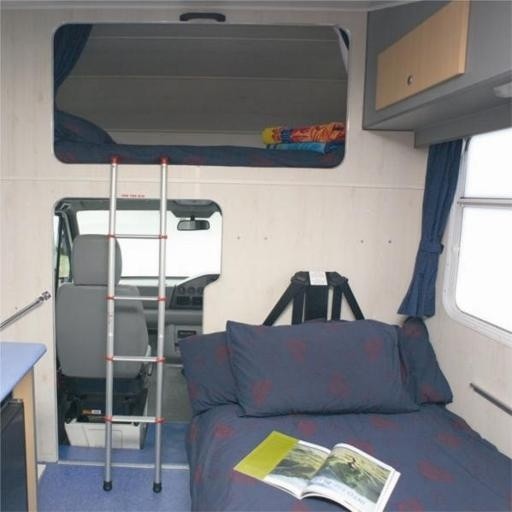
[0,342,47,512]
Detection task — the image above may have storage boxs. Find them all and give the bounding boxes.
[63,389,150,449]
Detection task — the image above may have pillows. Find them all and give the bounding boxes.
[226,320,419,417]
[177,331,238,417]
[399,317,453,405]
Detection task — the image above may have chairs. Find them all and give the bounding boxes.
[56,234,153,396]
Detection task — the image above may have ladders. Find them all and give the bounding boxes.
[103,154,169,493]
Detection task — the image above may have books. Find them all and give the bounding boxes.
[232,430,402,512]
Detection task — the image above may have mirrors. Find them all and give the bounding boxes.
[177,220,211,232]
[53,22,350,169]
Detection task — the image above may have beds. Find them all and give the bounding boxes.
[183,403,512,512]
